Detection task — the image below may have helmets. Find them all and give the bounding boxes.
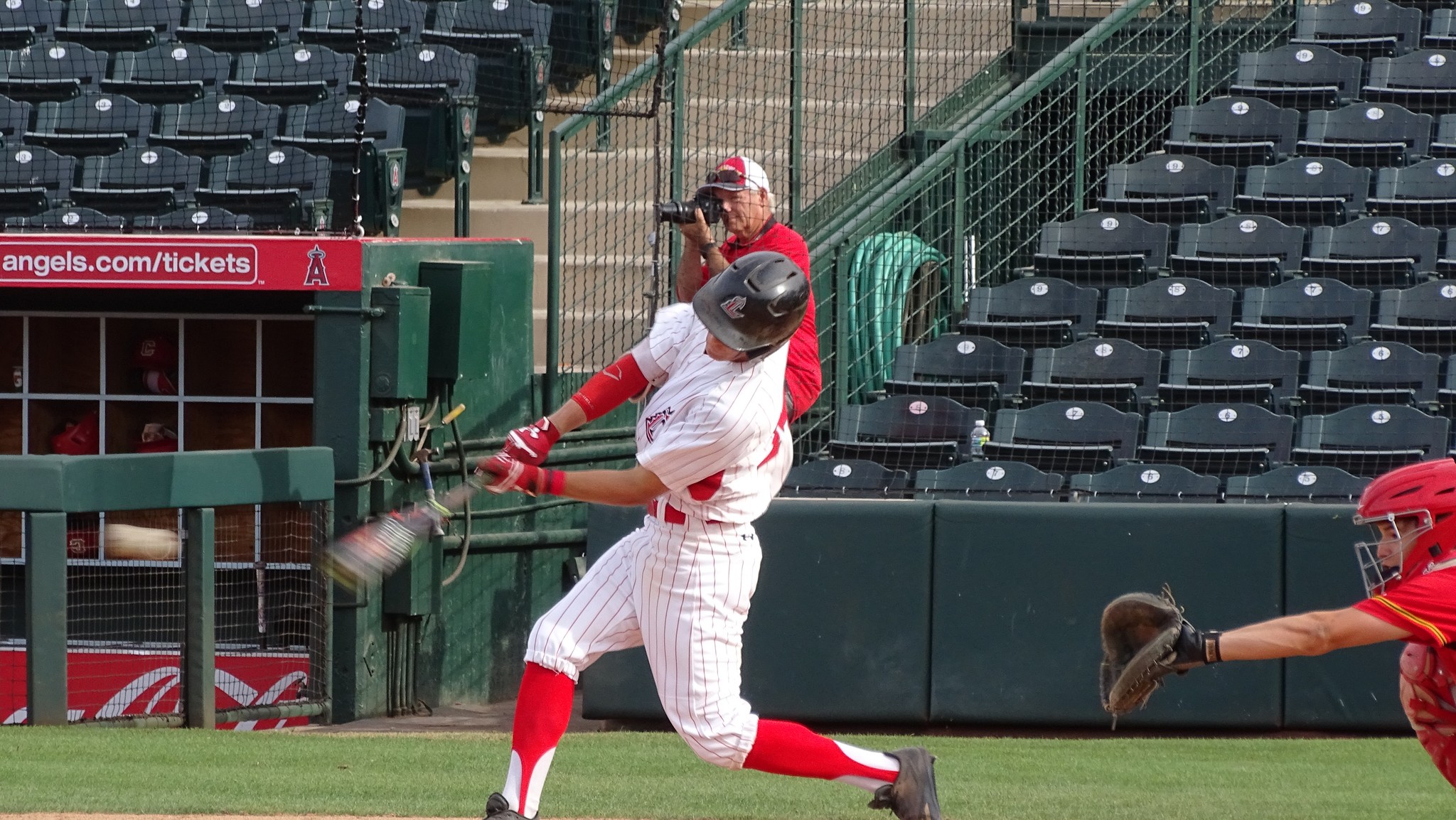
[1354,457,1456,597]
[691,250,809,351]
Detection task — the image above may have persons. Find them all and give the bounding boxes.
[1150,457,1456,790]
[51,329,184,560]
[477,252,942,820]
[676,156,822,426]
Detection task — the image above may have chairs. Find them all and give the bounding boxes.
[771,0,1455,508]
[0,0,670,234]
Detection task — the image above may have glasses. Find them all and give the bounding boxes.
[705,170,759,187]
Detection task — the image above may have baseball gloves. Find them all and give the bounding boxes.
[1100,583,1204,731]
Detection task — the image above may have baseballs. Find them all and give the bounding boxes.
[103,523,181,561]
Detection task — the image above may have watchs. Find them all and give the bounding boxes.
[700,242,717,259]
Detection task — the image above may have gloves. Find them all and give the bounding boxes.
[501,416,562,467]
[477,454,543,497]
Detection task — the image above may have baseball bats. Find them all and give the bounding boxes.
[315,472,496,598]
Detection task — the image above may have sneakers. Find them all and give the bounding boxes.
[869,748,943,820]
[485,793,540,820]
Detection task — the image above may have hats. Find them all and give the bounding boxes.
[697,156,769,194]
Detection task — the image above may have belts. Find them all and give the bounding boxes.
[646,499,722,524]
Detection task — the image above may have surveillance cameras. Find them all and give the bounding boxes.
[658,190,724,226]
